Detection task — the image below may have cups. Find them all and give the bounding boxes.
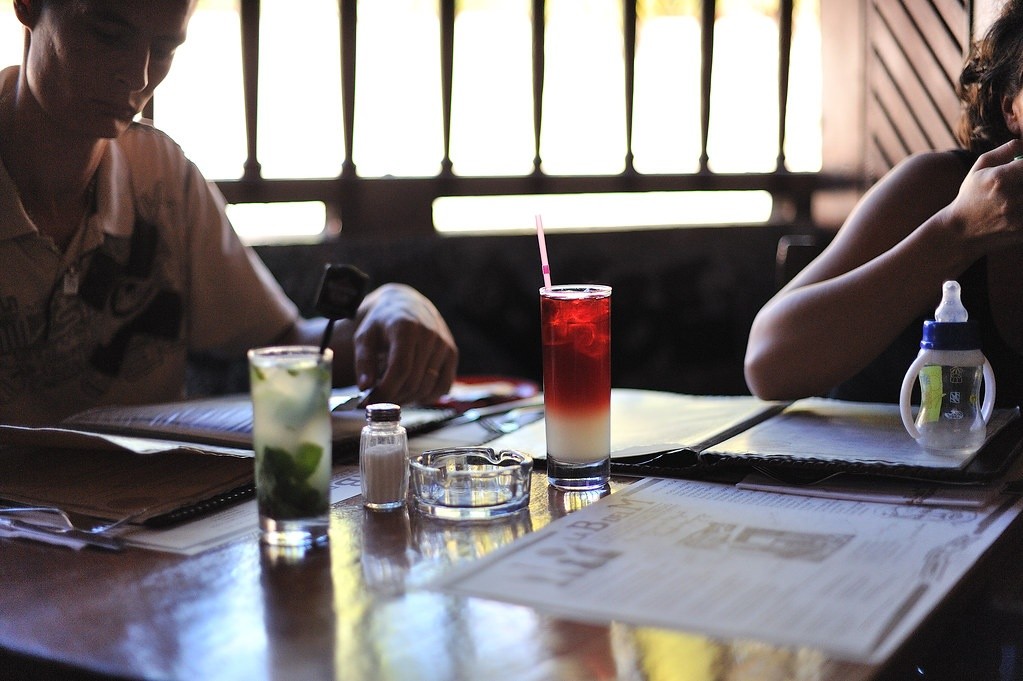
[247,346,332,547]
[540,284,612,491]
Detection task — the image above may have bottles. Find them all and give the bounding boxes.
[899,280,996,457]
[359,403,410,510]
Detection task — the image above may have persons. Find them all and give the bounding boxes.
[744,0,1023,402]
[0,0,458,450]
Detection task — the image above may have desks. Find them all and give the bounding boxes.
[0,400,1023,681]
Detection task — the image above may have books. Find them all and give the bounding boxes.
[0,384,458,460]
[485,387,1023,488]
